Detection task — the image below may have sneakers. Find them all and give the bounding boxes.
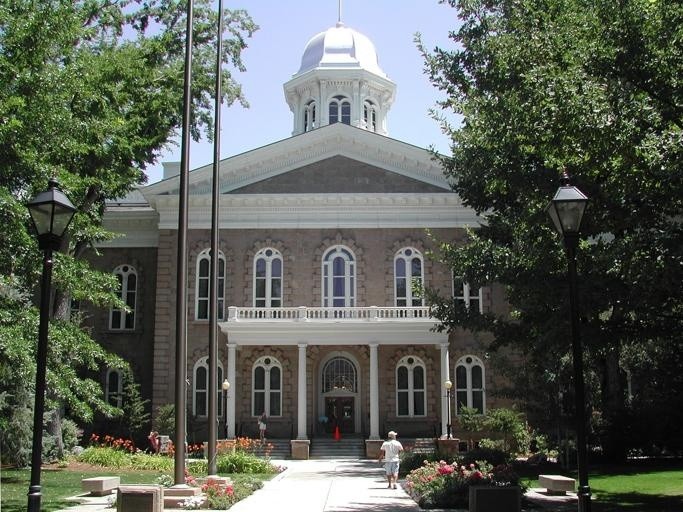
[388,485,397,489]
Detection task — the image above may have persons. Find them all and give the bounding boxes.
[257,410,267,442]
[377,430,403,488]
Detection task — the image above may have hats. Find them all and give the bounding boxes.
[388,431,397,438]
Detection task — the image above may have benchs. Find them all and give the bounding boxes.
[80,475,121,498]
[540,474,575,497]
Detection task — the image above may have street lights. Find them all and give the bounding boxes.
[24,173,80,512]
[544,164,591,512]
[221,378,231,440]
[444,378,453,440]
